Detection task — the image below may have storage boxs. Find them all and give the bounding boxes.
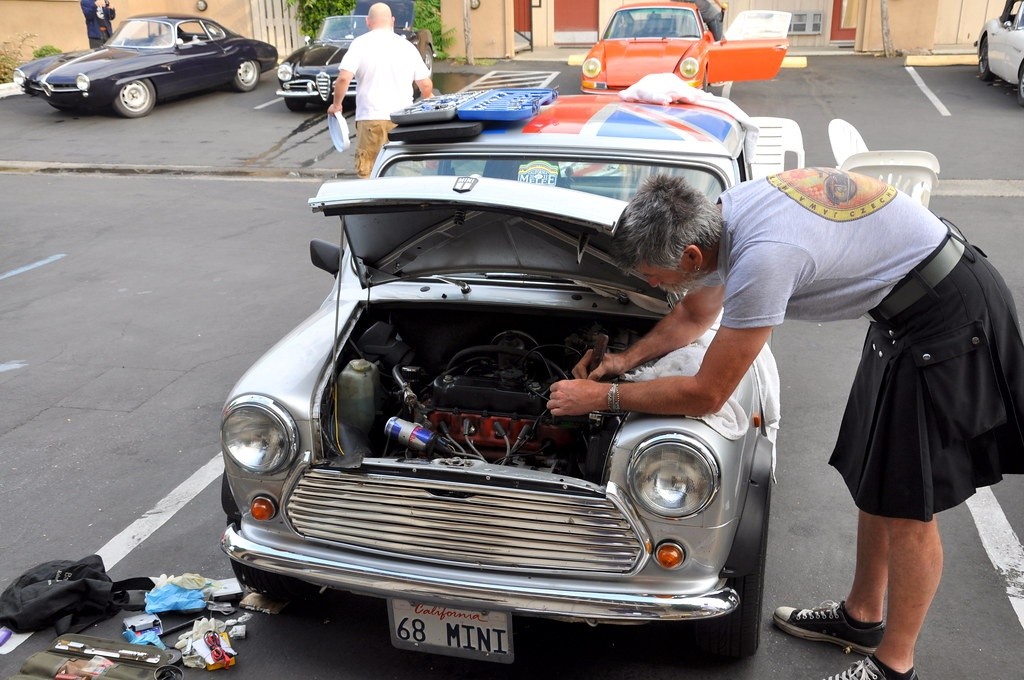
[388,87,557,121]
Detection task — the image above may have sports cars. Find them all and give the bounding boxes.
[13,13,278,118]
[276,0,434,111]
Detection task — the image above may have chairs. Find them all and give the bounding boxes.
[828,119,869,167]
[747,116,805,182]
[838,147,943,210]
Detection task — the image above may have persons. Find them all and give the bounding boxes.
[547,168,1024,680]
[686,0,723,41]
[81,0,115,48]
[327,3,434,179]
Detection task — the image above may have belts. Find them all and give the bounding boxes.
[863,220,966,323]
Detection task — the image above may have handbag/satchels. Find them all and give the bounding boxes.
[0,554,157,636]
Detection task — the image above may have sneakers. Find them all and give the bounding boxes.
[823,654,920,680]
[772,601,887,655]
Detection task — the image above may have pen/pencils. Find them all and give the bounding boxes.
[158,615,205,638]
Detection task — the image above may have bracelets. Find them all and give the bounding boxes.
[608,384,620,410]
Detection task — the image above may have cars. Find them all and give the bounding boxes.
[580,0,792,95]
[215,94,776,665]
[974,0,1024,107]
